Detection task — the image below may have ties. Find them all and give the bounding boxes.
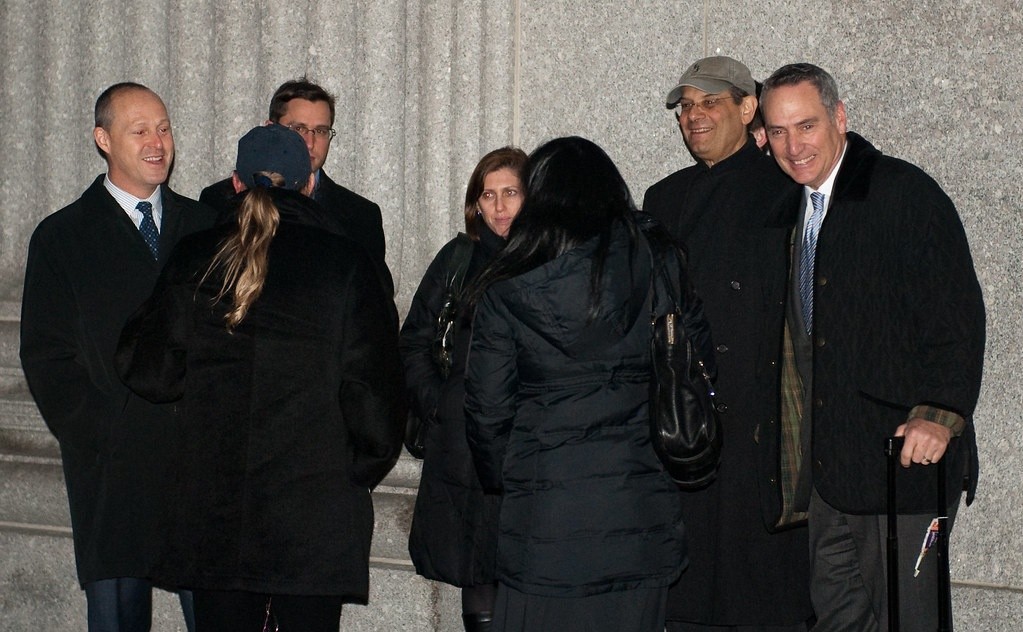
[799,192,825,338]
[135,201,159,263]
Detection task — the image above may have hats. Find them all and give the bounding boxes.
[666,55,756,105]
[236,125,311,193]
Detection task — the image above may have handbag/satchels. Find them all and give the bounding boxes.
[643,226,728,492]
[400,232,477,459]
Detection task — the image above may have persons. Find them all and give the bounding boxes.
[640,56,818,632]
[462,136,715,632]
[758,63,987,632]
[19,81,529,632]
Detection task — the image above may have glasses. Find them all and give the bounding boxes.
[675,97,734,117]
[288,125,335,141]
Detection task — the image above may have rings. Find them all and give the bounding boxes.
[923,456,931,463]
